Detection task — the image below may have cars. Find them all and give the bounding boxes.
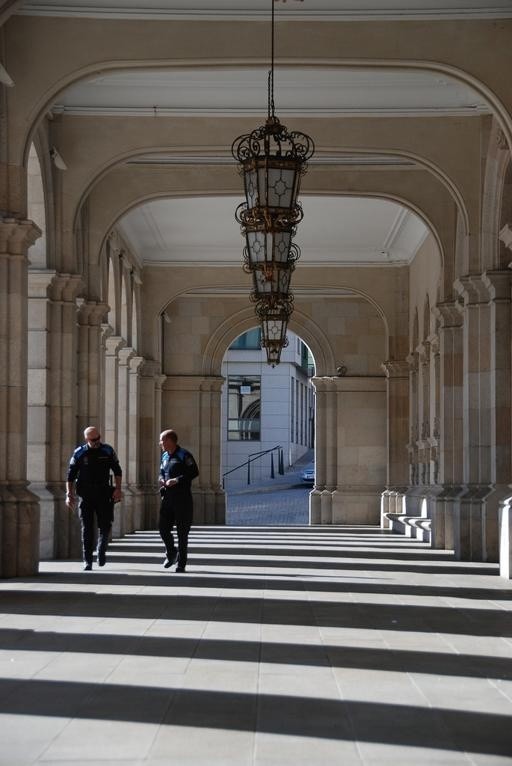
[299,459,314,482]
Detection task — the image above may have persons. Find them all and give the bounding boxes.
[64,427,122,571]
[156,427,200,574]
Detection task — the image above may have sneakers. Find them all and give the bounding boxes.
[163,547,186,573]
[84,547,106,571]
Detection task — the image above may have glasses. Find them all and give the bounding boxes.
[87,435,100,442]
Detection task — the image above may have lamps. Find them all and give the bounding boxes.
[230,0,313,366]
